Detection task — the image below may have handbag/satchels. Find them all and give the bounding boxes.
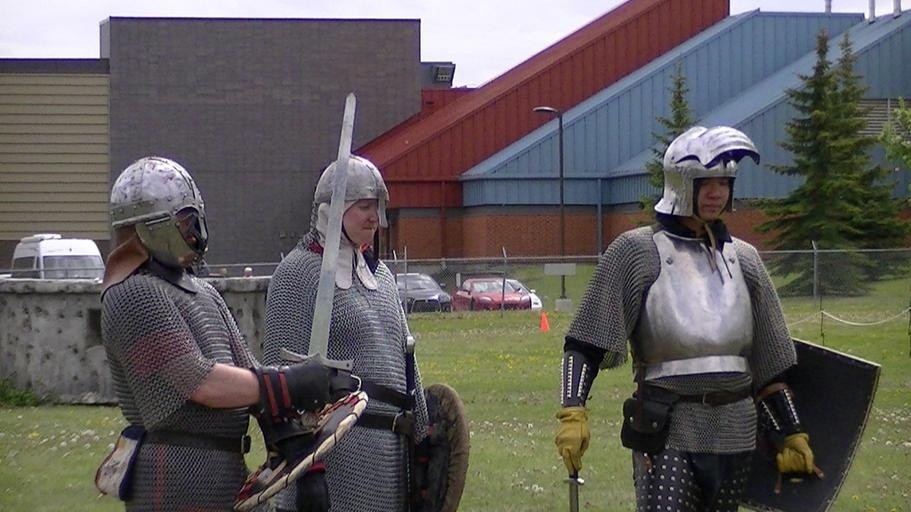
[619,399,673,455]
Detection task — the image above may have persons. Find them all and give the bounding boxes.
[263,155,431,512]
[94,156,337,512]
[555,126,814,512]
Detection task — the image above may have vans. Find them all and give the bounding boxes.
[10,234,105,279]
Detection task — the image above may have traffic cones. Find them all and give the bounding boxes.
[539,311,550,330]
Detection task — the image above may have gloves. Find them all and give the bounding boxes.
[776,434,816,484]
[554,407,591,474]
[295,464,331,511]
[250,360,330,419]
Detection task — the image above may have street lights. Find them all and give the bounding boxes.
[532,106,566,298]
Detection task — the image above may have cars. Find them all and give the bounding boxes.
[393,273,543,313]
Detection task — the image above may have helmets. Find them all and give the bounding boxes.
[654,127,761,217]
[109,156,207,270]
[310,154,389,233]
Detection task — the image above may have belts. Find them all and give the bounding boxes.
[359,383,418,433]
[676,383,752,407]
[144,430,251,455]
